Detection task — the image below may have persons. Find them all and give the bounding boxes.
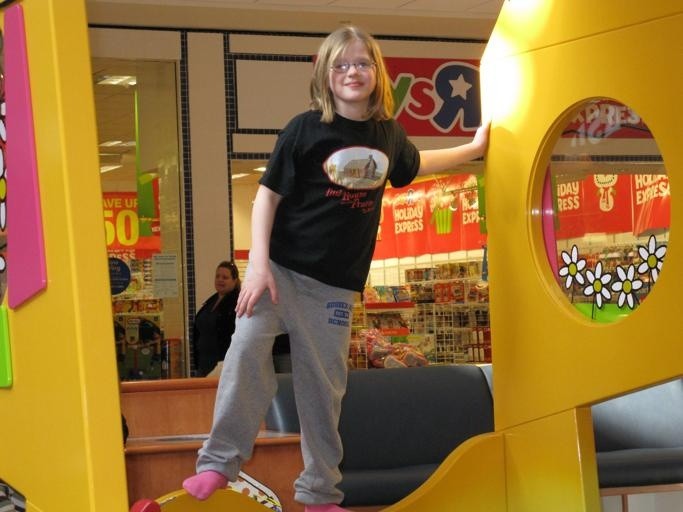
[182,24,493,512]
[194,260,240,375]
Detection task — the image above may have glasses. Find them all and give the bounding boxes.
[329,61,377,73]
